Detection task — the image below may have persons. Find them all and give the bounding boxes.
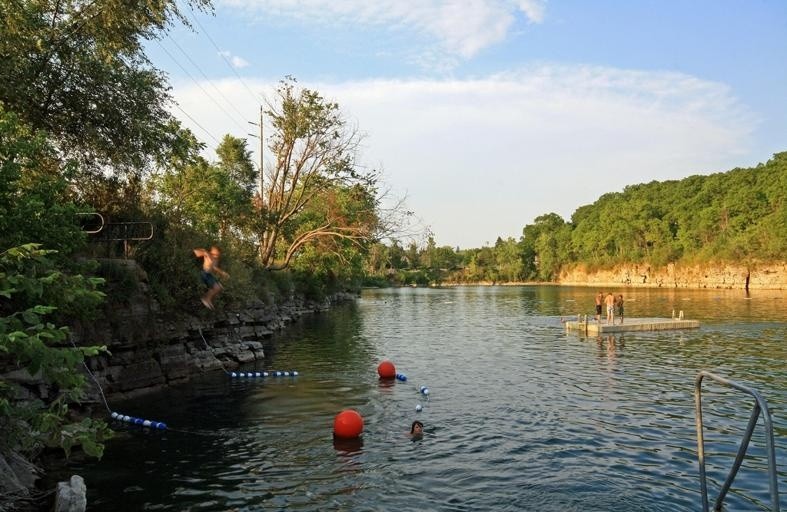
[410,421,423,437]
[604,293,616,324]
[193,246,229,309]
[617,295,624,324]
[595,292,604,323]
